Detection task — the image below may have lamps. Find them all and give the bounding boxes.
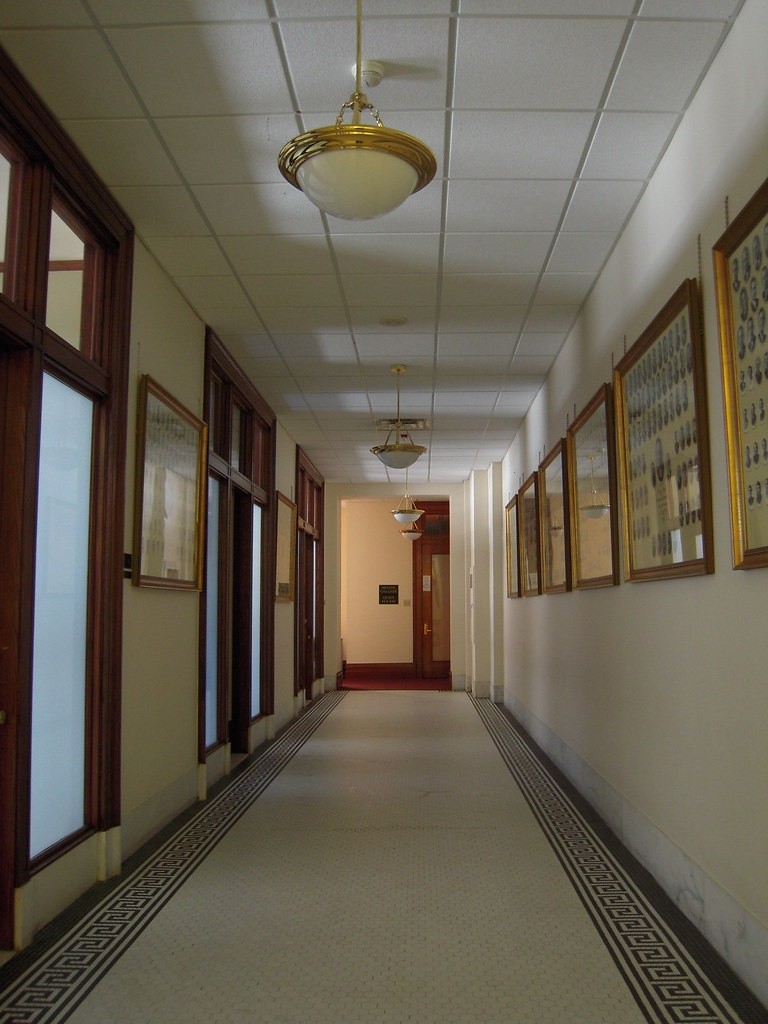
[276,0,438,222]
[399,521,424,540]
[391,492,425,523]
[370,363,427,471]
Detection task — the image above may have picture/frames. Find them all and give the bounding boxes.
[505,493,521,599]
[711,179,768,572]
[276,490,295,603]
[538,437,572,594]
[565,383,620,592]
[613,277,714,583]
[129,373,208,592]
[518,471,542,598]
